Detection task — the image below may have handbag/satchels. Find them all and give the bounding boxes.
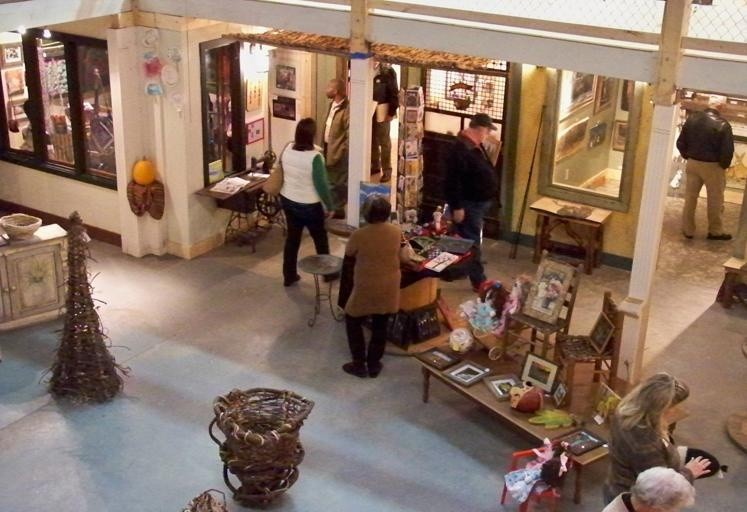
[400,234,414,264]
[261,142,290,196]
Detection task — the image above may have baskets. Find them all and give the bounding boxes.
[0,214,42,241]
[207,388,315,506]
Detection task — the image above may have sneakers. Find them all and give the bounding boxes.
[368,361,383,379]
[343,364,367,377]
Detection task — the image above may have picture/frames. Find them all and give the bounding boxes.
[480,372,523,402]
[6,97,30,123]
[550,426,609,459]
[586,310,615,352]
[439,359,492,390]
[518,350,561,396]
[553,383,567,405]
[412,343,463,372]
[1,41,24,70]
[556,65,637,164]
[521,258,575,326]
[243,117,266,144]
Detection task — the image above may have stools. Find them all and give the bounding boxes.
[299,252,347,326]
[722,256,746,309]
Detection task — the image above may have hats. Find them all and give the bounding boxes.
[678,445,727,485]
[471,112,498,130]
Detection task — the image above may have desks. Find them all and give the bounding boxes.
[376,231,477,357]
[528,195,612,274]
[408,314,693,506]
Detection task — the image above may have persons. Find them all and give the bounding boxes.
[602,466,696,512]
[676,95,734,240]
[278,117,340,286]
[320,78,350,219]
[443,114,501,293]
[603,372,712,506]
[371,61,399,184]
[338,195,406,378]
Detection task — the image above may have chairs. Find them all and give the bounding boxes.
[553,289,625,408]
[499,271,586,368]
[501,437,574,511]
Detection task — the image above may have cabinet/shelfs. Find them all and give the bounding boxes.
[0,222,80,332]
